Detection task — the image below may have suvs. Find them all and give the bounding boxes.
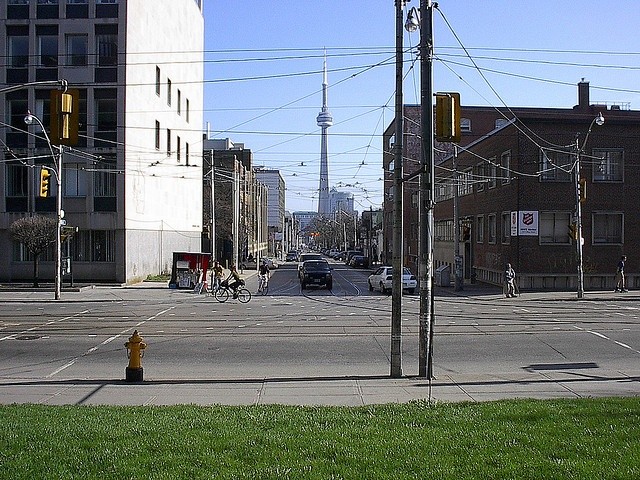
[297,253,321,277]
[329,249,339,258]
[345,251,361,265]
[300,259,333,289]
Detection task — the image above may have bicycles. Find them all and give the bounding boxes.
[209,273,225,292]
[257,275,271,296]
[215,281,251,303]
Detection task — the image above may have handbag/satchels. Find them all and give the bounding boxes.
[240,279,245,286]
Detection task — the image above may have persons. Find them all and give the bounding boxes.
[614,254,629,292]
[502,262,518,297]
[221,265,242,298]
[249,252,256,263]
[209,262,224,288]
[256,261,270,291]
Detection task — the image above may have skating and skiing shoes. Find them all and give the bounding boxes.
[622,286,629,294]
[614,286,621,293]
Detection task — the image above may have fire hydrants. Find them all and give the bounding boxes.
[124,330,146,382]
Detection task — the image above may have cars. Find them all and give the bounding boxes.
[325,250,329,255]
[333,251,347,261]
[240,258,276,270]
[367,266,418,293]
[286,253,297,261]
[350,255,369,269]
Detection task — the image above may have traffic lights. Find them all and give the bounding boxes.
[460,226,470,241]
[567,224,577,241]
[40,169,52,197]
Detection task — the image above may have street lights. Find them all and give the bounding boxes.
[336,210,357,247]
[24,109,66,299]
[405,7,436,377]
[574,111,605,298]
[328,219,347,252]
